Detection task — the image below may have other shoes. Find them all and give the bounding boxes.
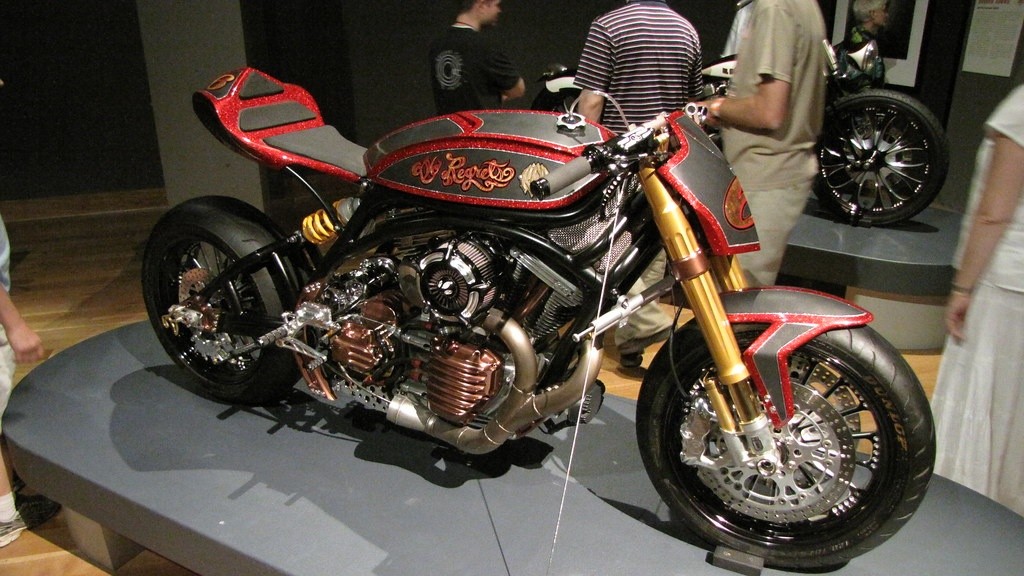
[617,326,671,354]
[603,345,645,366]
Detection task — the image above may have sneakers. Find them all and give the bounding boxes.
[11,470,26,492]
[0,491,62,548]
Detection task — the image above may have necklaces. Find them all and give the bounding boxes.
[455,21,478,30]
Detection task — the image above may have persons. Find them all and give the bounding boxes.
[928,80,1024,522]
[852,0,889,44]
[428,0,525,118]
[0,216,60,548]
[698,0,827,288]
[575,0,703,366]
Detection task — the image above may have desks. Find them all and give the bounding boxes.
[1,315,1024,576]
[774,192,966,350]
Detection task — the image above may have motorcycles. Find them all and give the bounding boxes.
[531,34,951,226]
[139,63,936,572]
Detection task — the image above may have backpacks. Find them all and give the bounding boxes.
[827,31,885,105]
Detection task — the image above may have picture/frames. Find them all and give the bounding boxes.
[831,0,930,88]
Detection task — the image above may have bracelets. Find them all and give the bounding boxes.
[950,283,972,295]
[710,97,724,117]
